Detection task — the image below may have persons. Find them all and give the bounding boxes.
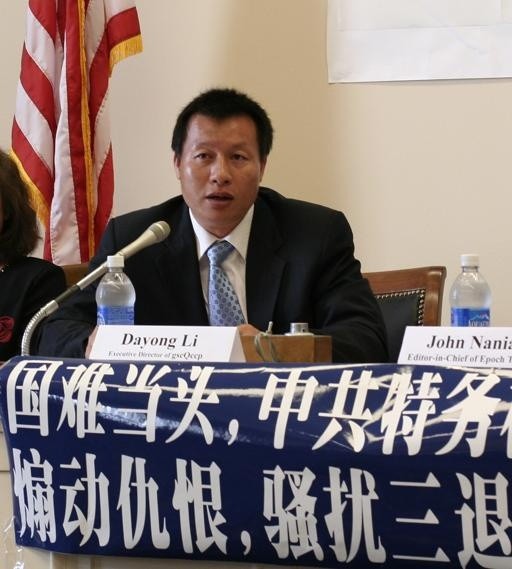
[0,145,65,359]
[32,86,394,365]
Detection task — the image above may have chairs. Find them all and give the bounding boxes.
[361,266,448,362]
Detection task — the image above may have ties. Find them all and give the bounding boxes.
[206,240,247,326]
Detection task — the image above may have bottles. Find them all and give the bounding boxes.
[449,253,491,327]
[95,255,136,325]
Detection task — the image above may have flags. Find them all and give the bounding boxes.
[10,0,145,265]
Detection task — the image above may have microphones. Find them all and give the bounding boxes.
[21,220,170,357]
